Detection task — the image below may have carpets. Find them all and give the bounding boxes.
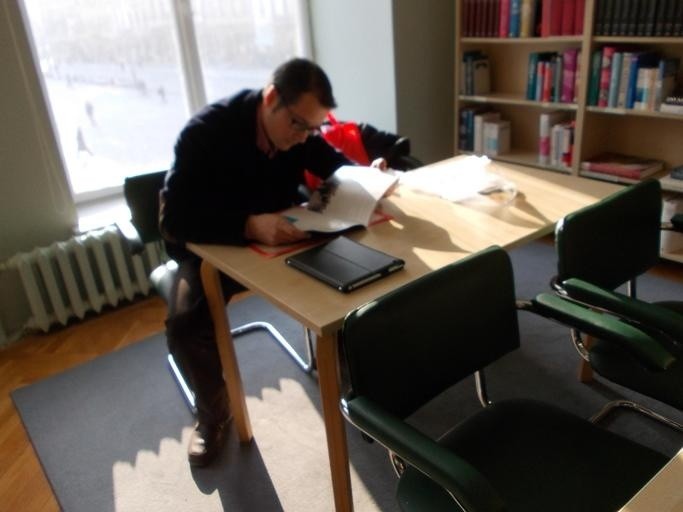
[12,239,683,512]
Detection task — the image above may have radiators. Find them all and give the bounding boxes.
[6,224,159,332]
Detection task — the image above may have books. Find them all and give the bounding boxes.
[659,196,682,264]
[589,45,683,114]
[538,108,576,168]
[278,164,399,235]
[458,104,511,158]
[594,1,683,36]
[461,48,491,94]
[579,154,683,189]
[527,47,582,103]
[458,1,585,38]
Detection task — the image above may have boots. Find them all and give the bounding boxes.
[187,386,231,467]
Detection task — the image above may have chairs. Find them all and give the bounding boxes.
[337,245,673,511]
[549,179,683,412]
[123,170,316,415]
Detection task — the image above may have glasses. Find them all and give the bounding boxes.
[288,109,322,137]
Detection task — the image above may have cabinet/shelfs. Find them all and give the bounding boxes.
[454,0,683,263]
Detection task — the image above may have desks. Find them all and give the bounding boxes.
[617,447,683,512]
[187,153,627,512]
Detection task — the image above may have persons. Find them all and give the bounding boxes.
[157,60,396,463]
